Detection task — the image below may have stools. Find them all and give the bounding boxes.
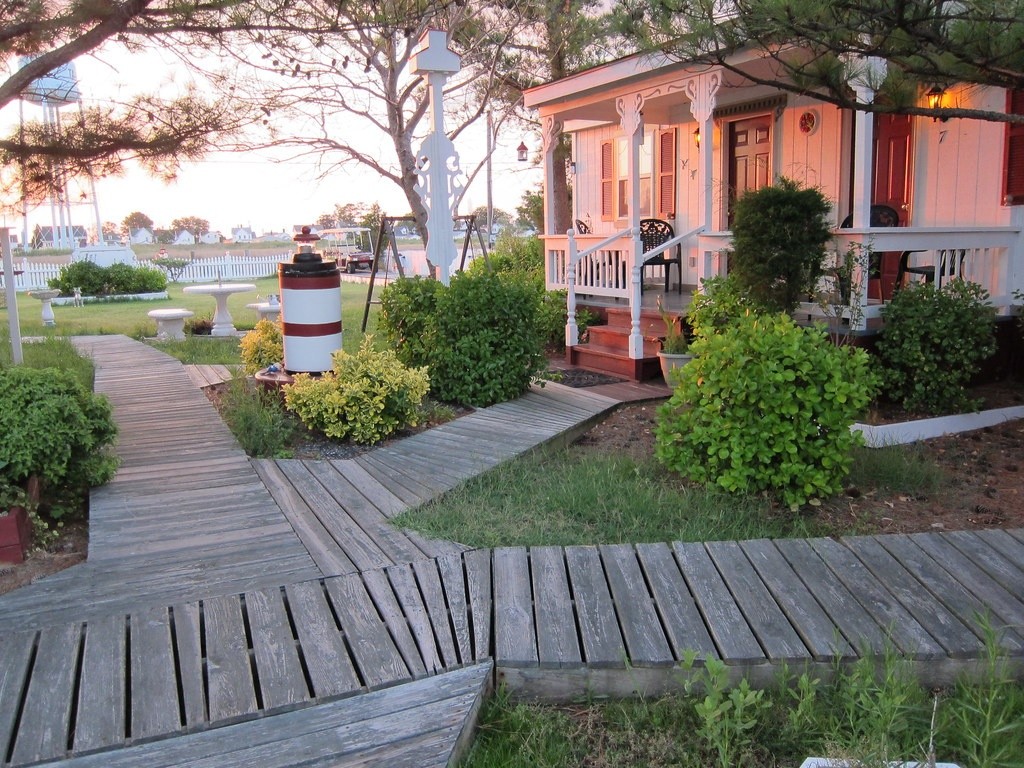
[148,308,193,342]
[248,300,282,326]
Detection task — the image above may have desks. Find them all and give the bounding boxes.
[184,284,256,336]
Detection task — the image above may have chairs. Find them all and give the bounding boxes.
[889,249,967,304]
[630,219,682,296]
[807,204,899,326]
[575,219,627,300]
